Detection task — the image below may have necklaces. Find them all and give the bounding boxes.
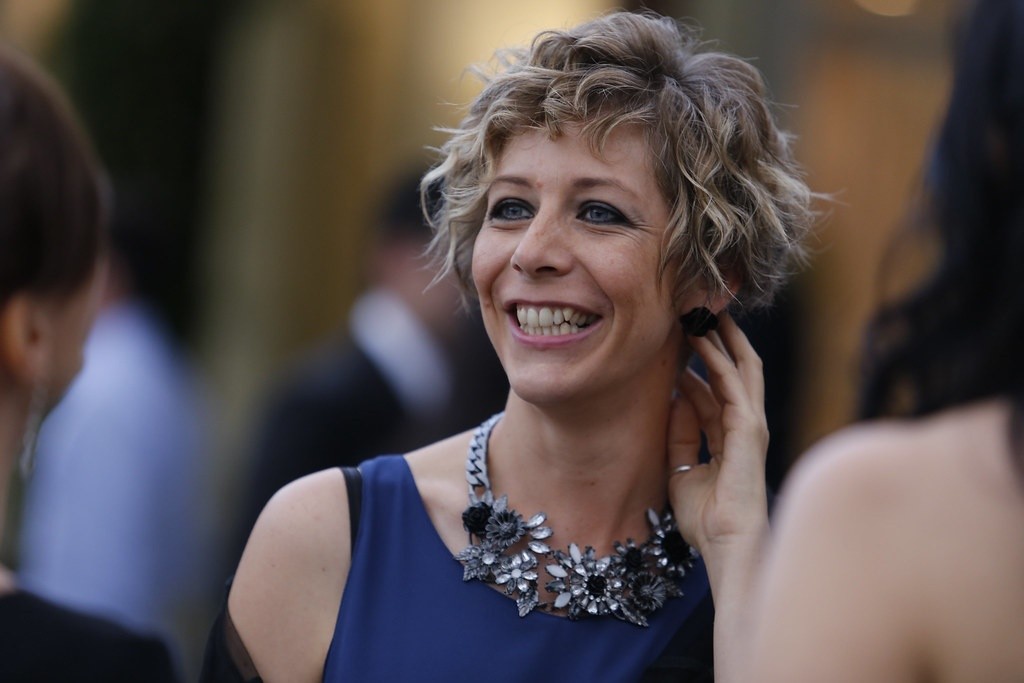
[454,409,696,626]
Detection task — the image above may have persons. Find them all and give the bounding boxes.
[206,157,508,683]
[199,6,813,683]
[0,39,187,683]
[0,139,210,683]
[739,2,1024,683]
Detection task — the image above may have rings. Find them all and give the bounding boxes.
[666,464,694,477]
